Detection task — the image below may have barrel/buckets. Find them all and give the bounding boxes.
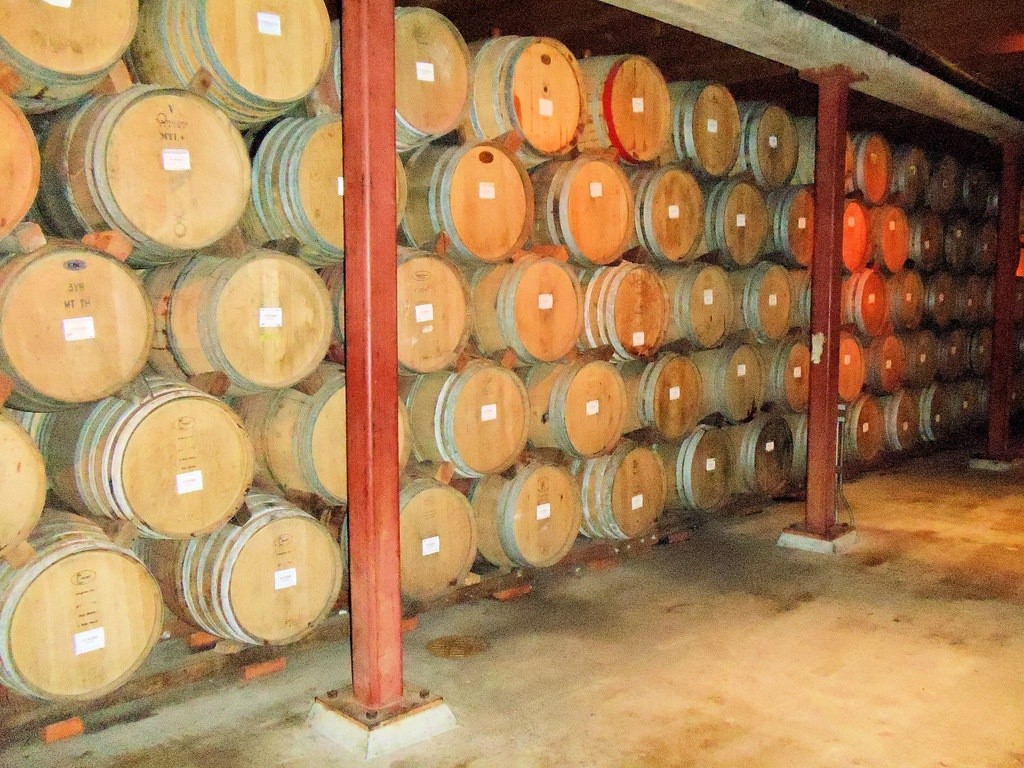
[0,0,1001,701]
